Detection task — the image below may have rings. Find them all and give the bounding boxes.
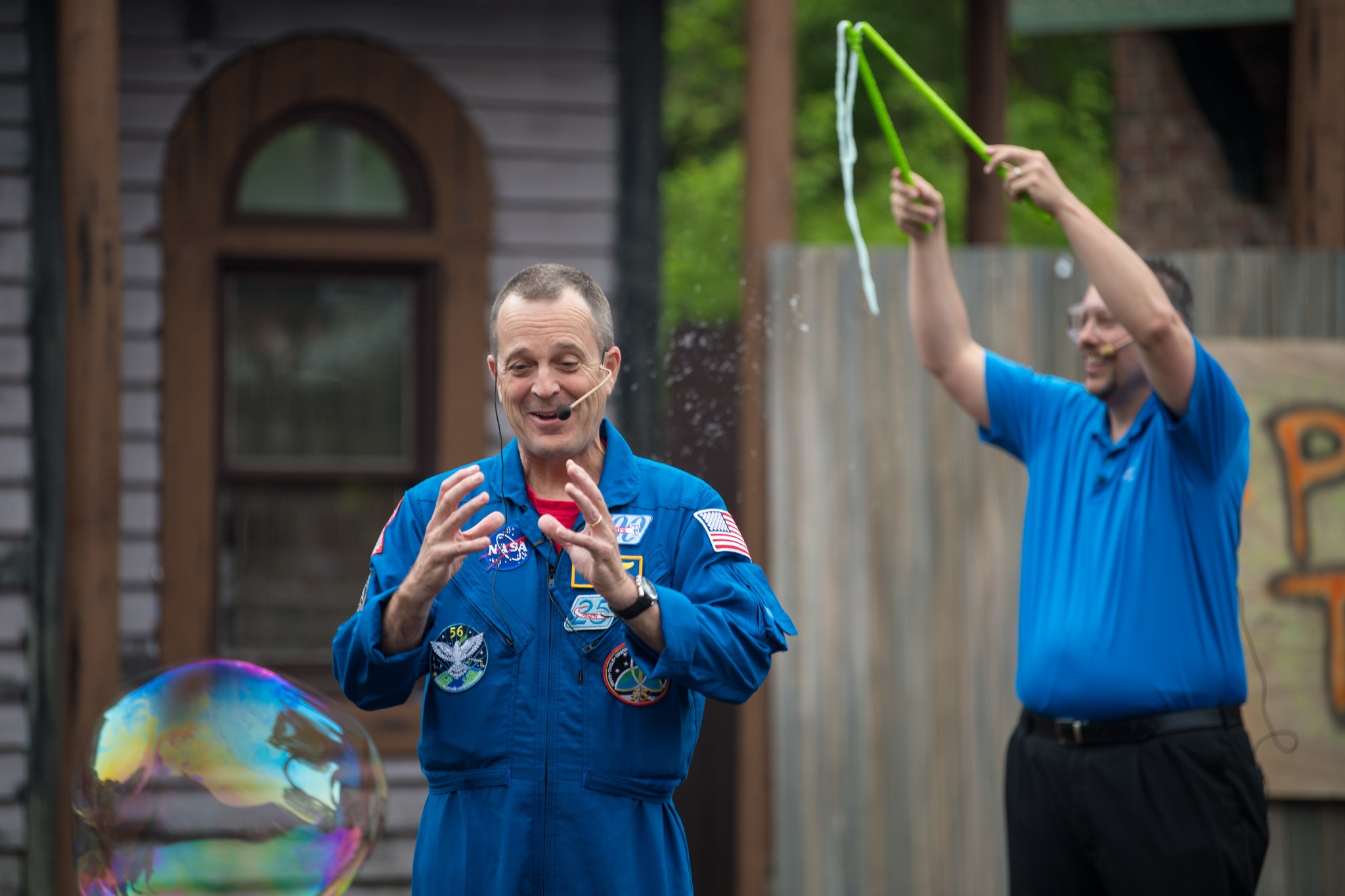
[1015,167,1022,178]
[590,514,602,528]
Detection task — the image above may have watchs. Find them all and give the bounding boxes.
[607,575,658,621]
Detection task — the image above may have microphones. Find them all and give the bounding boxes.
[1100,335,1135,357]
[555,346,612,420]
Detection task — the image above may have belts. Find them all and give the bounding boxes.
[1026,710,1228,745]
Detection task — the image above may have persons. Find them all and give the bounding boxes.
[886,142,1271,896]
[330,262,799,896]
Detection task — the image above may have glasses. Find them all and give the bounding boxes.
[1064,304,1131,344]
